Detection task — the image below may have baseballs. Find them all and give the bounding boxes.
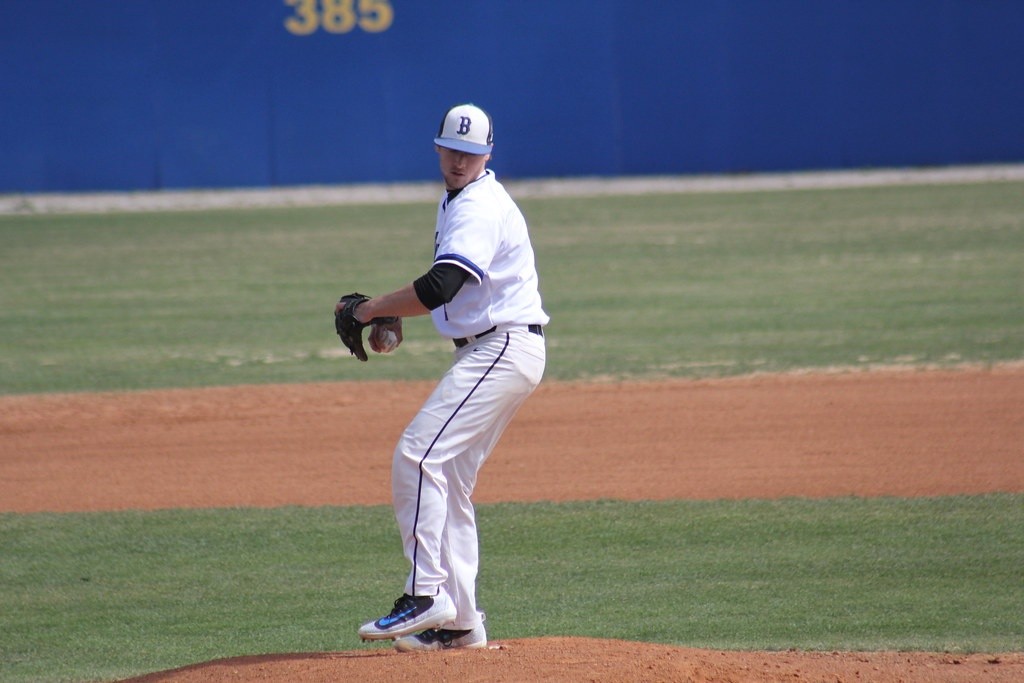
[381,331,398,354]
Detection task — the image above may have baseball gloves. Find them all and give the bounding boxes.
[331,292,371,361]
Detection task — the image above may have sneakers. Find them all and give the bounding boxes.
[393,611,487,651]
[357,582,457,643]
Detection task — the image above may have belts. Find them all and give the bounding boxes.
[453,324,543,348]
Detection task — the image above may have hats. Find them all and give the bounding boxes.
[434,102,494,155]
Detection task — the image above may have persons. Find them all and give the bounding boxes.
[334,102,550,654]
